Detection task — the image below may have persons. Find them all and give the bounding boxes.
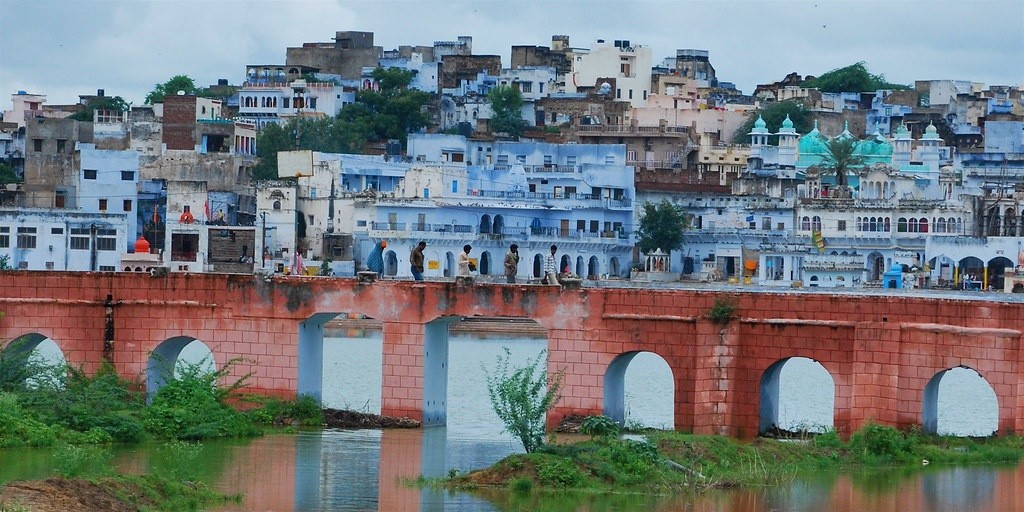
[218,209,225,226]
[247,255,252,264]
[503,244,519,283]
[457,244,472,275]
[239,252,246,262]
[409,241,426,280]
[367,241,387,280]
[544,245,558,285]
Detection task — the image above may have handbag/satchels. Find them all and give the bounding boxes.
[541,279,548,284]
